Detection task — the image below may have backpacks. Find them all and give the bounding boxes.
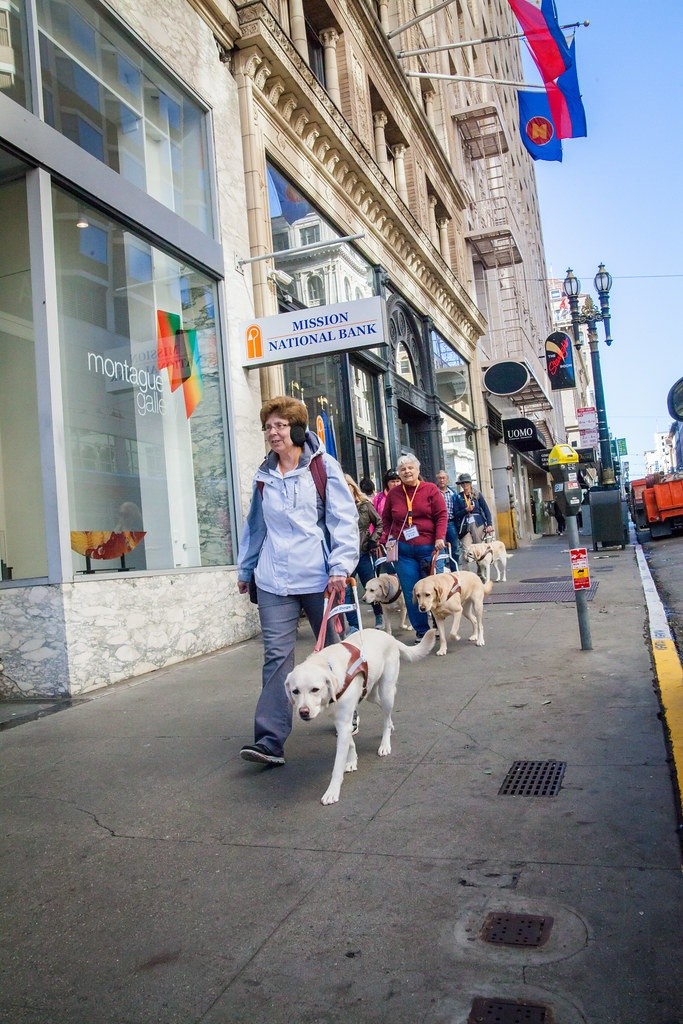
[549,501,555,515]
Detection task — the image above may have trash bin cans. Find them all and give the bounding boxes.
[541,500,557,535]
[590,491,625,541]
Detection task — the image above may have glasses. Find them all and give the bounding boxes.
[262,422,292,432]
[387,470,399,476]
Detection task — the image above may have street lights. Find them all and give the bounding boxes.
[564,262,617,489]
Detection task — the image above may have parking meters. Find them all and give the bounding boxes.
[549,444,593,648]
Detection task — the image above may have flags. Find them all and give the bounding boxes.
[507,0,588,162]
[157,309,203,419]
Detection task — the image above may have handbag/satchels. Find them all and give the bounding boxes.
[249,573,258,603]
[385,539,398,563]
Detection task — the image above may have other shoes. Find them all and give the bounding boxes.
[436,635,439,639]
[414,634,424,644]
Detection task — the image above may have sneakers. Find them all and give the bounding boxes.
[336,710,359,736]
[346,626,358,636]
[375,614,385,628]
[239,744,285,765]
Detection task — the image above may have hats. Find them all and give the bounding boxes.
[456,474,475,483]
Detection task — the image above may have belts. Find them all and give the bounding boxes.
[448,519,454,521]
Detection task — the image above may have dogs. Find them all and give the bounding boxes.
[412,571,494,656]
[285,628,436,805]
[465,541,515,583]
[362,573,407,636]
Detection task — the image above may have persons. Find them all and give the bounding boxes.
[235,395,361,764]
[343,452,582,645]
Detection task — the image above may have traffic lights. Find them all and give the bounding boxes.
[613,461,621,476]
